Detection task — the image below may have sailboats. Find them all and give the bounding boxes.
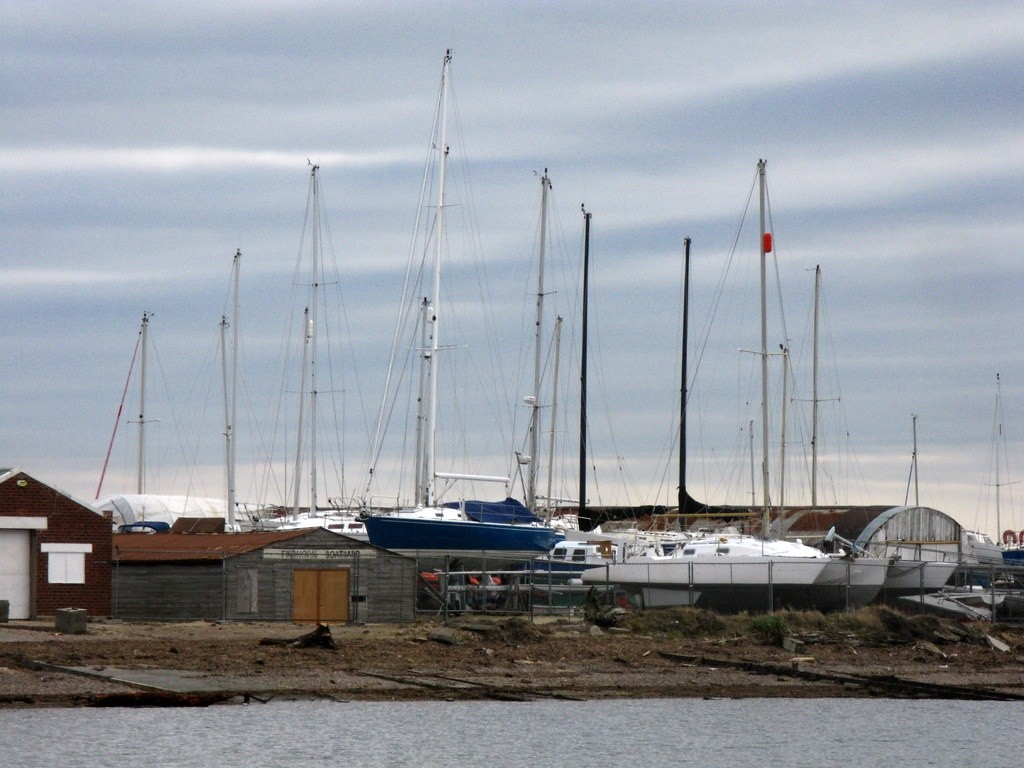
[355,46,567,558]
[89,159,1024,596]
[582,159,900,615]
